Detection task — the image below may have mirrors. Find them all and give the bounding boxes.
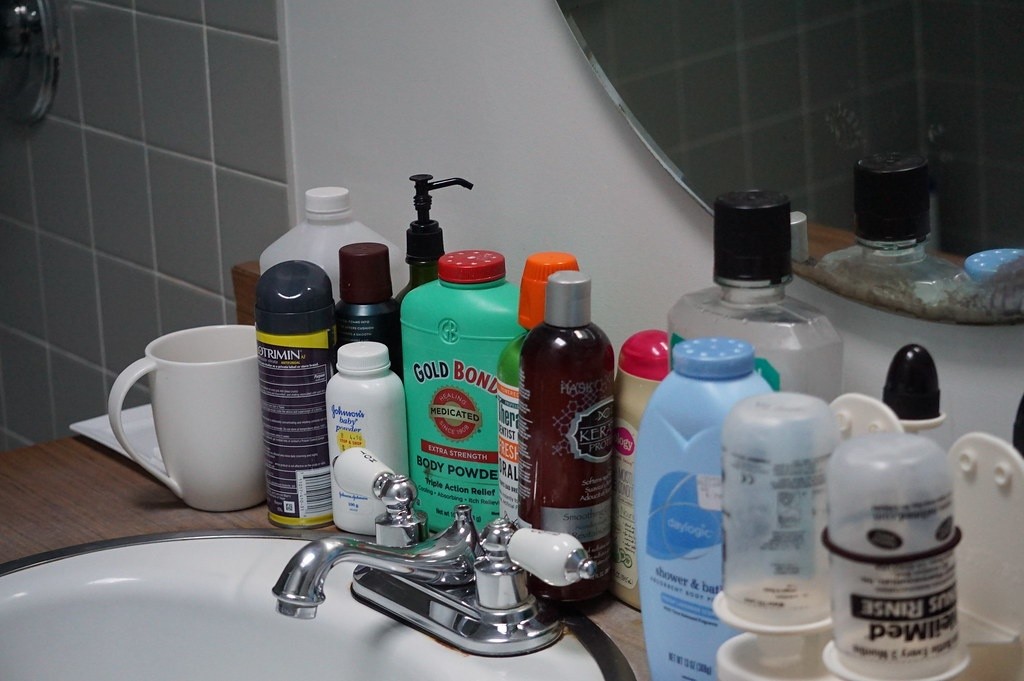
[551,0,1024,326]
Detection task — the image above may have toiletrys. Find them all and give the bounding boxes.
[255,166,1024,679]
[779,151,1024,307]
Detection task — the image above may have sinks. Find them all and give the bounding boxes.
[1,531,608,681]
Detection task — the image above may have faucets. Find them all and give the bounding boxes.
[265,446,598,658]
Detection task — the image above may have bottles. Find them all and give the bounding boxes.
[259,185,410,303]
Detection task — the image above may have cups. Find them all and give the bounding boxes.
[108,326,266,512]
